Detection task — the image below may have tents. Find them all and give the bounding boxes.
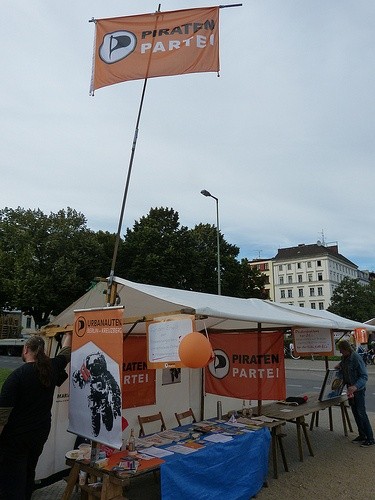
[34,275,375,488]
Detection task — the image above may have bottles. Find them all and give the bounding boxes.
[241,400,247,417]
[128,428,136,452]
[248,400,253,418]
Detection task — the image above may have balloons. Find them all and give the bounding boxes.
[178,332,212,368]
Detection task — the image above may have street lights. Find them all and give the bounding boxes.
[200,189,221,296]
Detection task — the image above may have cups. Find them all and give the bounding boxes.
[78,471,87,486]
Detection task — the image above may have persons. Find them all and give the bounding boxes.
[362,348,375,366]
[333,340,375,447]
[0,332,72,500]
[289,342,295,359]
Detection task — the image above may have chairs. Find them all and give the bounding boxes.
[176,407,195,427]
[136,413,168,434]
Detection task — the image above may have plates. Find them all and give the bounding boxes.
[65,450,80,459]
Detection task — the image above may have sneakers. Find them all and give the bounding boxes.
[361,439,375,447]
[351,436,368,443]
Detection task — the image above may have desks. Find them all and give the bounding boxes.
[237,393,354,463]
[61,413,286,500]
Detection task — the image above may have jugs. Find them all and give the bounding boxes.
[78,443,91,462]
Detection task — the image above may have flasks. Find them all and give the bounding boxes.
[217,401,222,421]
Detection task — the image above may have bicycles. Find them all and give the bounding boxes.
[283,345,301,360]
